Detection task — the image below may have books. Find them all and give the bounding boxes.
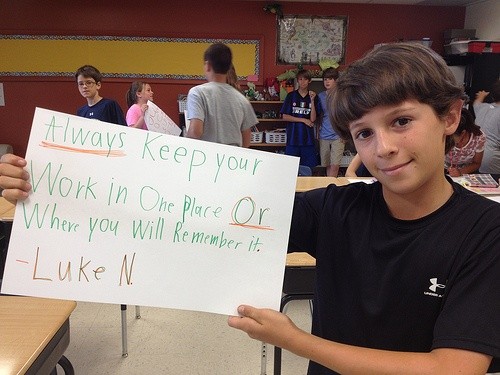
[464,174,499,195]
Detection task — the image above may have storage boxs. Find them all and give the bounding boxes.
[450,40,500,54]
[280,87,294,100]
[467,41,500,53]
[442,45,450,56]
[443,29,476,44]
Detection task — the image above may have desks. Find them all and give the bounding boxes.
[178,112,186,137]
[0,176,500,375]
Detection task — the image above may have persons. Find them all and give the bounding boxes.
[186,43,259,148]
[0,42,500,375]
[345,151,374,178]
[317,67,345,178]
[281,70,323,177]
[473,80,500,174]
[125,80,154,131]
[445,109,485,177]
[76,65,128,126]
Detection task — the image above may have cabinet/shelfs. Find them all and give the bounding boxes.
[249,99,320,154]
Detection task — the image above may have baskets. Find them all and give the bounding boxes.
[179,101,188,112]
[264,129,287,143]
[340,153,355,167]
[249,131,263,143]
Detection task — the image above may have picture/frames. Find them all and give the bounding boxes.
[276,14,347,66]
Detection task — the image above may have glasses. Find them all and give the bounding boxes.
[78,81,96,86]
